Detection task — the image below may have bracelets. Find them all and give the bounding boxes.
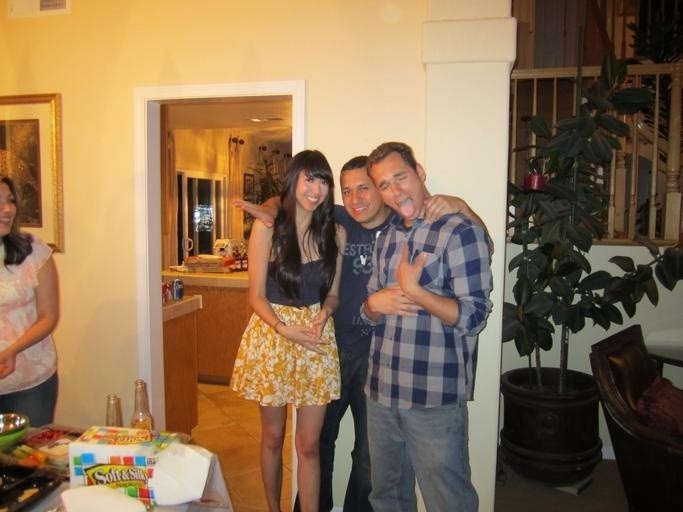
[274,321,285,334]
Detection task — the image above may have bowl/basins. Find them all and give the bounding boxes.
[0,413,30,452]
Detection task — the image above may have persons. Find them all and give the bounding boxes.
[0,176,59,429]
[230,149,347,512]
[359,143,495,512]
[232,156,494,512]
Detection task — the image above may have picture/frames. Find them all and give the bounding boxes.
[0,94,63,253]
[244,174,254,194]
[245,199,254,219]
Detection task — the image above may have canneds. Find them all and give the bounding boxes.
[172,279,183,301]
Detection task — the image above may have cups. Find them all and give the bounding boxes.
[524,173,549,190]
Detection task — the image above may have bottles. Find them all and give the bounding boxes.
[235,248,248,271]
[105,379,155,430]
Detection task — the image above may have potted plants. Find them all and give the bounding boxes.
[500,53,683,483]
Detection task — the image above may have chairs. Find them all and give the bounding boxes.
[588,324,683,512]
